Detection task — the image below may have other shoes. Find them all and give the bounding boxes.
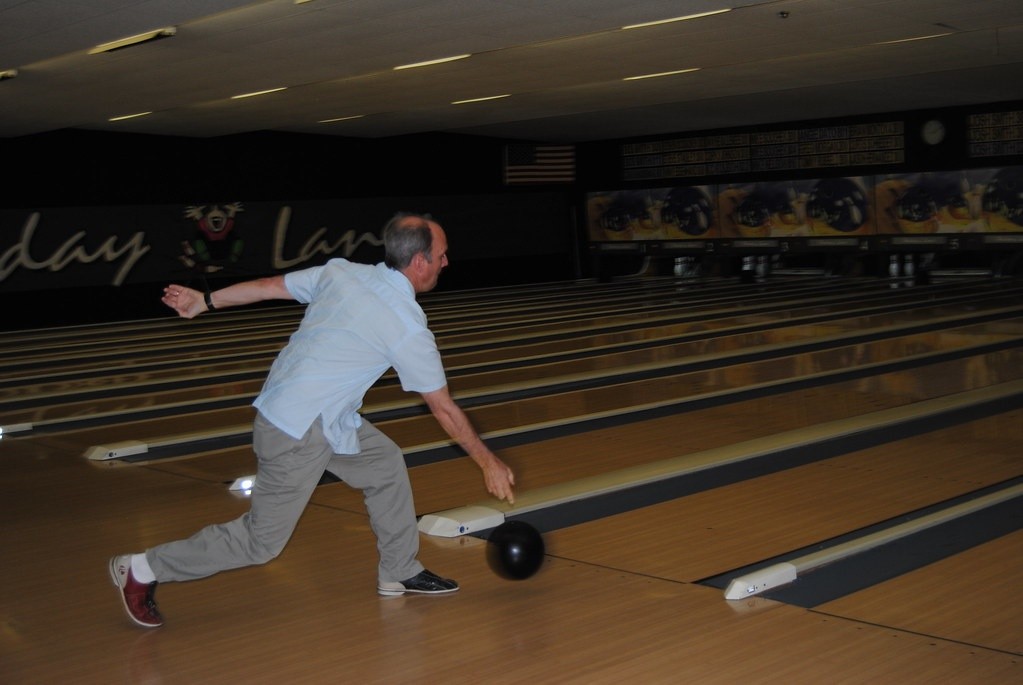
[109,553,164,626]
[377,568,460,596]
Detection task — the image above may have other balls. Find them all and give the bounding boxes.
[485,520,544,581]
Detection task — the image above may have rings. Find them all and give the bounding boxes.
[176,291,179,296]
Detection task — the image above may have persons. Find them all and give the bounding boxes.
[108,214,517,627]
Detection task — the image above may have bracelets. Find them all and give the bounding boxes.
[204,291,215,310]
